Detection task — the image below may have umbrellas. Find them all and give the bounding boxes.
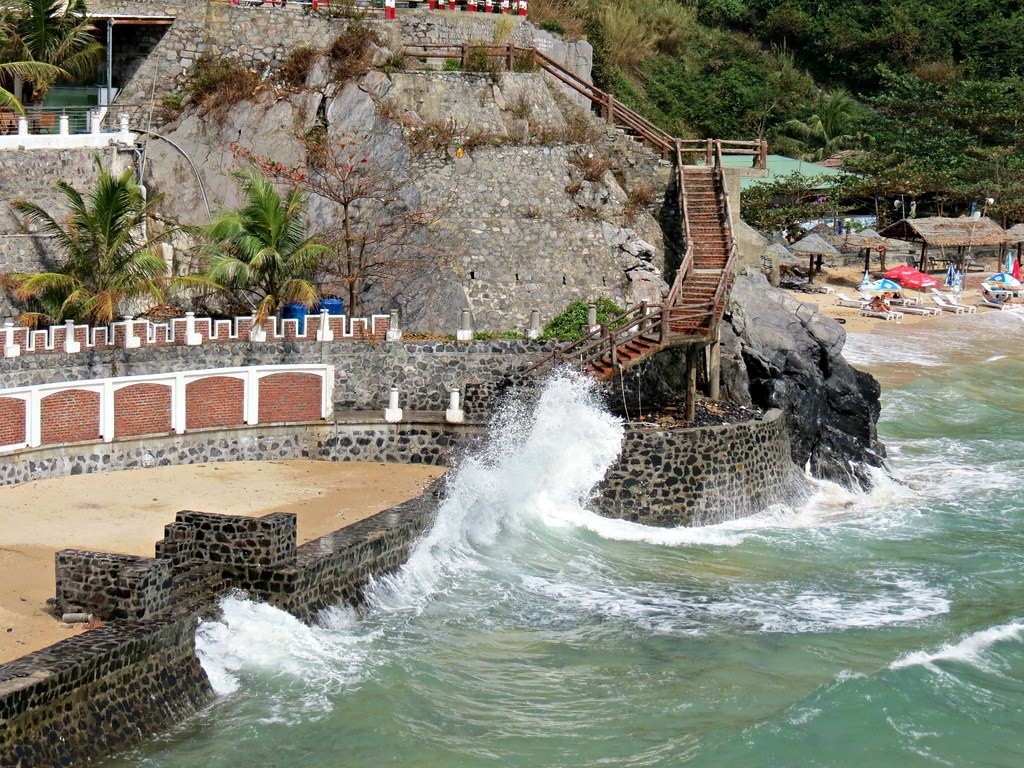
[981,272,1021,286]
[859,270,871,301]
[884,265,940,299]
[787,222,847,282]
[1006,223,1024,266]
[1005,252,1022,280]
[943,263,963,291]
[759,243,801,285]
[848,228,888,273]
[866,278,901,294]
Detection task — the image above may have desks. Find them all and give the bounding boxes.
[934,260,950,271]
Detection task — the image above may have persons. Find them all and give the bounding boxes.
[869,296,890,314]
[884,292,899,299]
[982,290,1011,304]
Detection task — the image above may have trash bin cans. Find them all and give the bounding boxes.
[315,298,342,315]
[283,303,308,334]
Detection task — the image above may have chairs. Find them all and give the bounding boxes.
[819,256,1024,321]
